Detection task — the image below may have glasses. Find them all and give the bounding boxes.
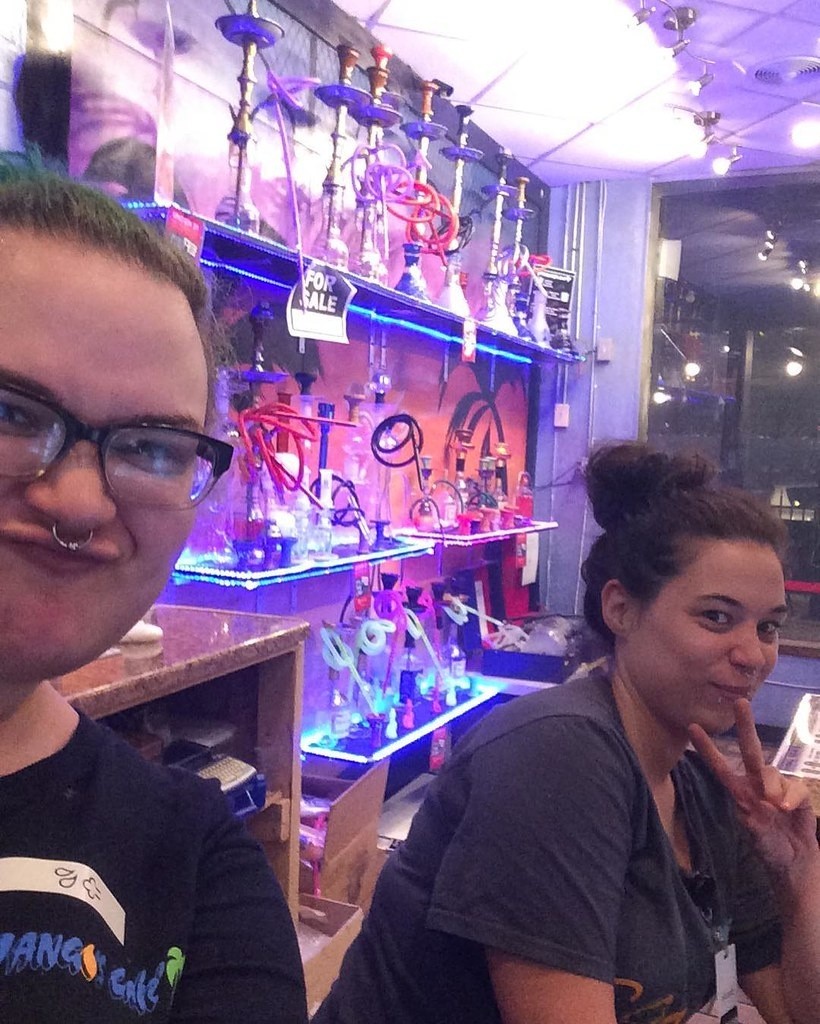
[0,383,235,511]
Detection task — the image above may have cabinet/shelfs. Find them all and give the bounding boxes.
[125,200,580,765]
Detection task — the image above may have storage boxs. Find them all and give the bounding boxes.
[299,892,364,1019]
[301,756,391,905]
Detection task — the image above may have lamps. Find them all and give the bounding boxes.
[622,0,743,176]
[758,228,777,260]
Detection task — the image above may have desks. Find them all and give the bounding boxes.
[50,605,312,938]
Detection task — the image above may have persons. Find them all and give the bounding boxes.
[0,162,309,1024]
[308,440,820,1024]
